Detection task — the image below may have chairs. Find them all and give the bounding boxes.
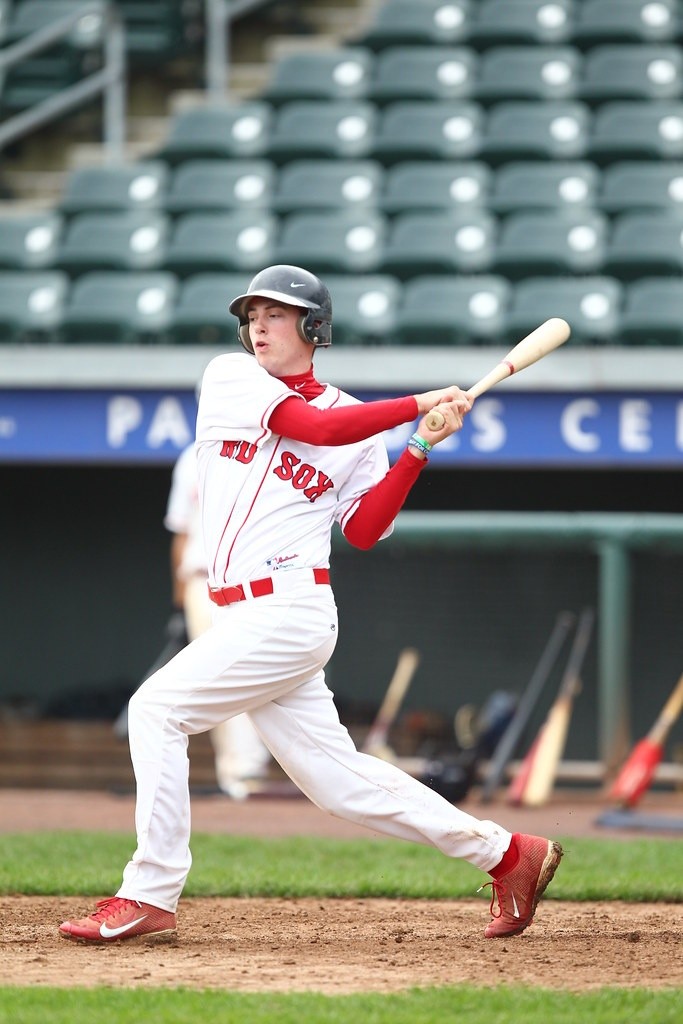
[0,0,683,352]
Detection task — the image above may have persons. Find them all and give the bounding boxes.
[162,374,270,804]
[55,261,562,945]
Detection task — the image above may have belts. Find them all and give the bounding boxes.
[207,568,330,606]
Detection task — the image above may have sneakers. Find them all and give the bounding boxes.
[476,831,562,938]
[58,898,177,944]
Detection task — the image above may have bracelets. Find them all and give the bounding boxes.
[407,434,432,455]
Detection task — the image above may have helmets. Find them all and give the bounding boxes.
[228,265,331,355]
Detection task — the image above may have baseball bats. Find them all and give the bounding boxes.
[521,597,596,809]
[607,675,683,807]
[424,317,571,431]
[504,718,547,806]
[358,650,419,756]
